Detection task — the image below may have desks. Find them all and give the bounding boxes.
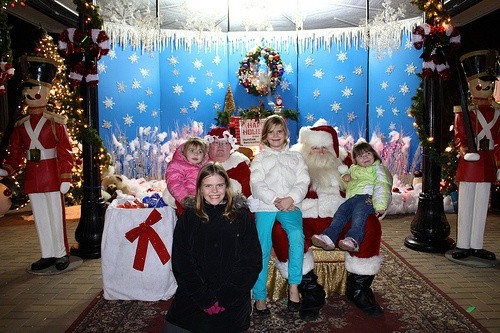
[102,199,178,301]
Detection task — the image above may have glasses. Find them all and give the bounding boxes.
[212,139,229,147]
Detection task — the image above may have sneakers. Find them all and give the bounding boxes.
[338,237,359,252]
[312,234,335,251]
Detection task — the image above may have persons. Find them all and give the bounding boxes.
[451,48,500,261]
[0,55,76,270]
[164,115,395,321]
[163,164,262,333]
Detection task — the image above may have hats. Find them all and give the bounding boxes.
[204,127,239,155]
[299,126,348,175]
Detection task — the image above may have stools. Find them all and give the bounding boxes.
[266,246,348,303]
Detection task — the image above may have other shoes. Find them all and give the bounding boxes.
[254,302,272,318]
[288,288,301,312]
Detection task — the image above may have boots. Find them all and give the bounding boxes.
[275,249,327,322]
[344,250,384,317]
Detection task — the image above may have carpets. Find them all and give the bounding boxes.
[62,238,491,333]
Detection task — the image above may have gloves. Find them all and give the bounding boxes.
[203,301,225,315]
[180,196,196,210]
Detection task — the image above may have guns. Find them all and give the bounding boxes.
[450,47,477,154]
